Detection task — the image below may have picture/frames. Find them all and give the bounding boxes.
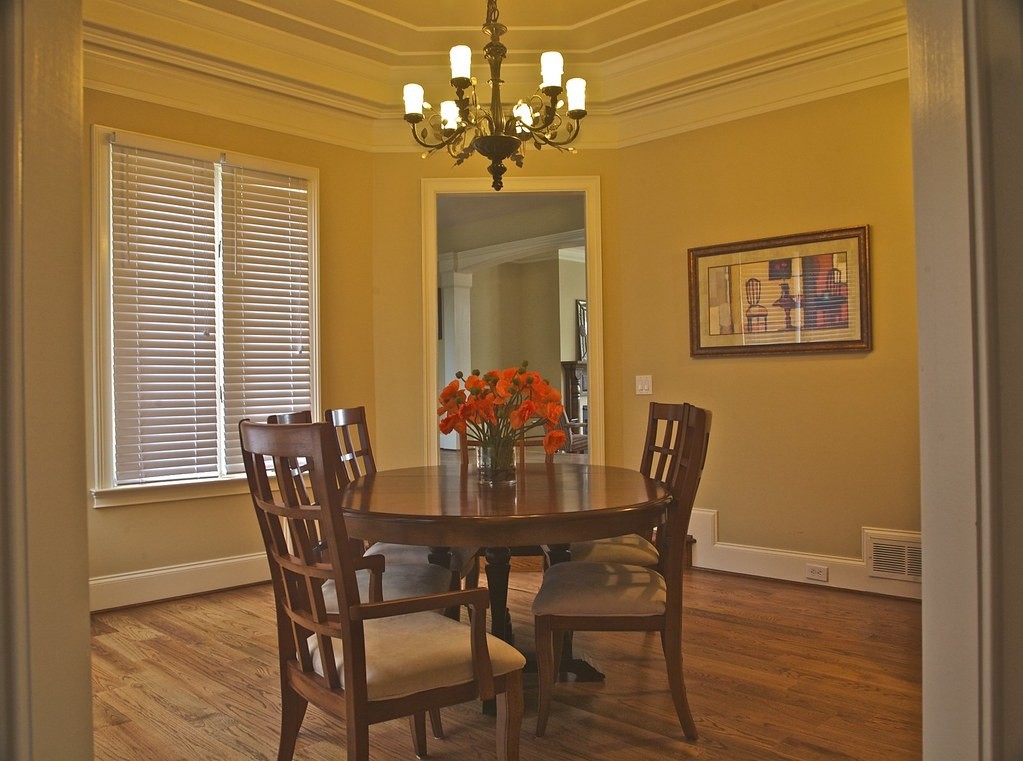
[686,224,873,360]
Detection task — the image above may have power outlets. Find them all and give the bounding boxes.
[805,563,828,582]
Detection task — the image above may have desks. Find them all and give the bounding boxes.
[331,463,676,718]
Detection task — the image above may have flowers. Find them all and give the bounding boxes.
[437,360,568,477]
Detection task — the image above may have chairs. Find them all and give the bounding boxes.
[238,417,527,761]
[325,405,480,628]
[533,401,714,738]
[267,411,455,741]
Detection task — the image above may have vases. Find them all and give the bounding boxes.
[471,414,517,485]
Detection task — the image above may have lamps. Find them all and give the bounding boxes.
[403,0,588,192]
